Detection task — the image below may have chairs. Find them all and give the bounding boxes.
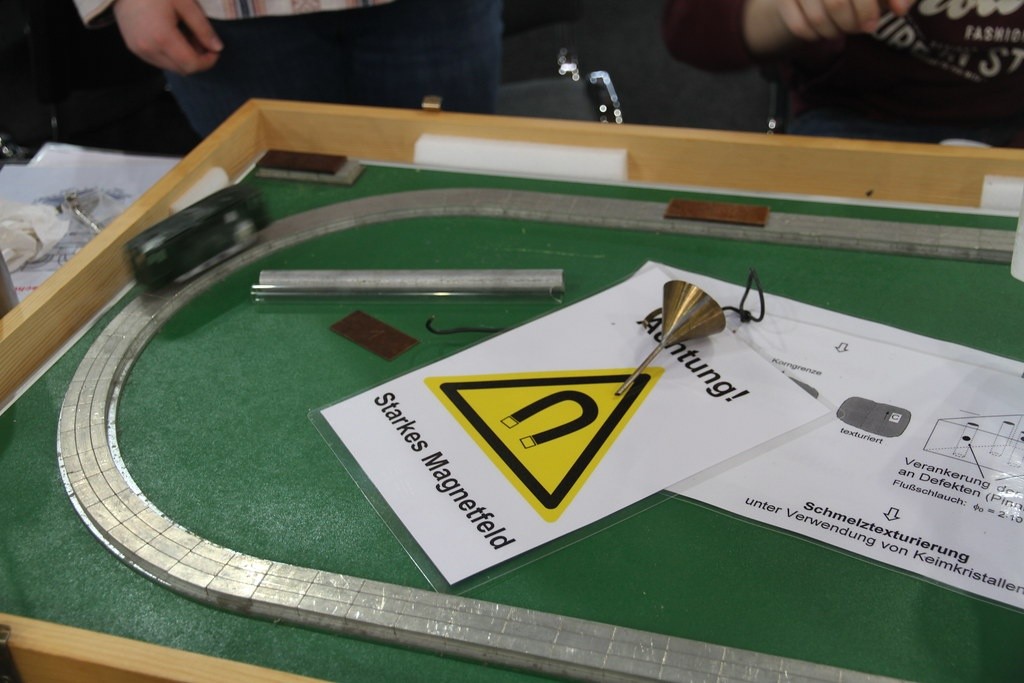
[493,70,624,125]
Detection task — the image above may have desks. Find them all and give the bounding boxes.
[0,141,182,319]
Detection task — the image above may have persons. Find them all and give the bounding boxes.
[661,1,1024,150]
[71,0,505,138]
